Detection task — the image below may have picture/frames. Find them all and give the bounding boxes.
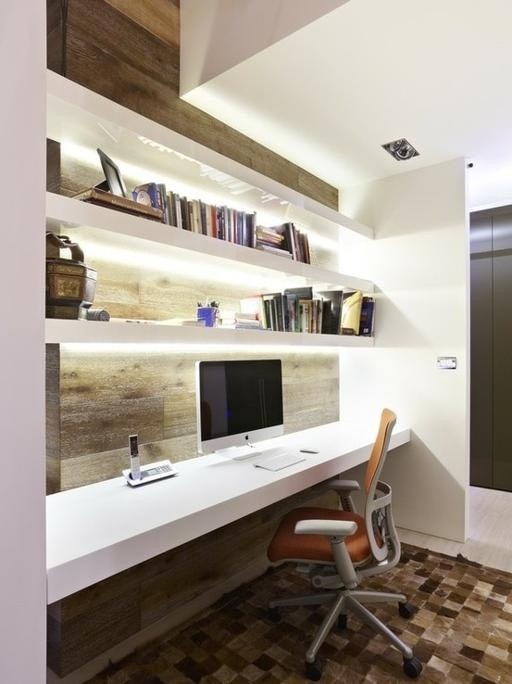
[95,148,127,198]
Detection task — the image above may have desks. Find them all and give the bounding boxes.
[46,418,411,684]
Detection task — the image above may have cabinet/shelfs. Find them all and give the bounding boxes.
[46,69,377,353]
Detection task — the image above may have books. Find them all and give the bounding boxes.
[70,183,310,264]
[235,287,375,337]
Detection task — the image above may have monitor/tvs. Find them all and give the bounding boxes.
[194,358,284,463]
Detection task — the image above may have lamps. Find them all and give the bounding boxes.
[381,138,419,161]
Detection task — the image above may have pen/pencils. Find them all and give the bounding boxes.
[198,296,220,308]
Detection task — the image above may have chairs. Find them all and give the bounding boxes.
[268,408,422,677]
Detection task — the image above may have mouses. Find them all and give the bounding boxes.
[299,448,320,453]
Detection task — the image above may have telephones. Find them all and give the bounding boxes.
[122,435,177,486]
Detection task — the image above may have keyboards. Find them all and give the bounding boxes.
[254,451,306,472]
